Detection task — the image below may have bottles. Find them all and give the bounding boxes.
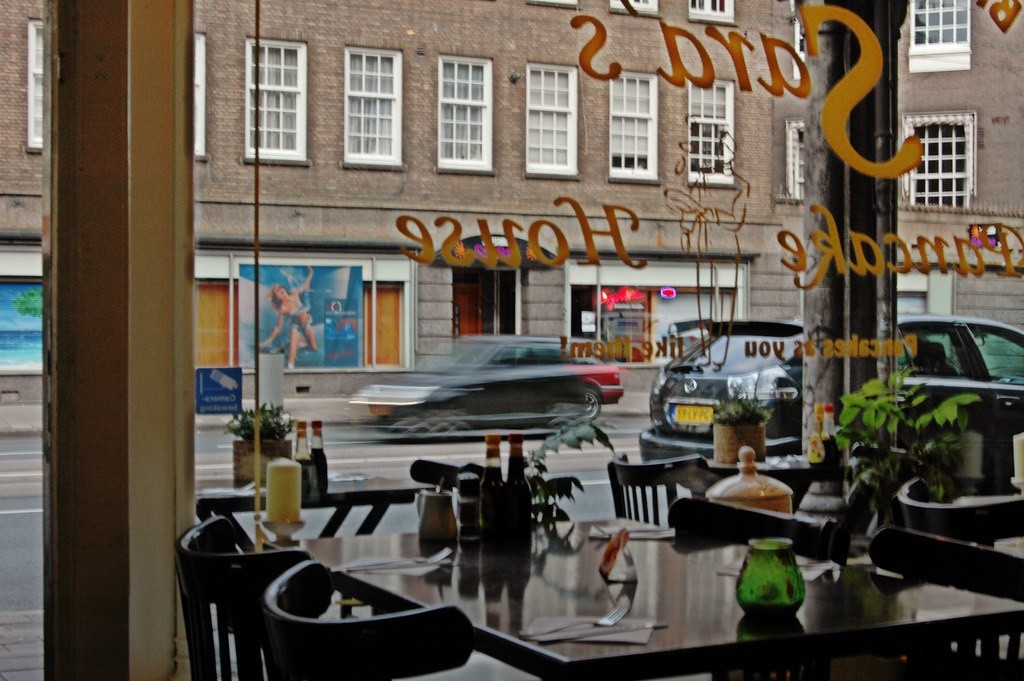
[457,433,533,555]
[737,539,806,618]
[293,420,331,498]
[806,399,840,468]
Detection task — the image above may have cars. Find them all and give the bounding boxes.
[564,358,624,424]
[369,337,602,438]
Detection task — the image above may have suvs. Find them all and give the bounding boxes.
[636,310,1024,500]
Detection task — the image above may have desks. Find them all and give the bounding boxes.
[704,448,853,484]
[198,468,567,543]
[220,514,1024,681]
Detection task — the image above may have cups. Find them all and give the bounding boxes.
[414,492,458,544]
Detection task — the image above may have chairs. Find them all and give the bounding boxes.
[177,442,1024,681]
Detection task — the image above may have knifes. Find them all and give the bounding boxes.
[538,622,669,642]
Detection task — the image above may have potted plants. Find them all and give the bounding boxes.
[837,370,970,534]
[716,398,766,462]
[231,403,297,489]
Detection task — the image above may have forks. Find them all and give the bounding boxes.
[517,596,630,638]
[343,547,452,563]
[593,524,671,534]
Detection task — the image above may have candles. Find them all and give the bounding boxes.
[267,455,300,521]
